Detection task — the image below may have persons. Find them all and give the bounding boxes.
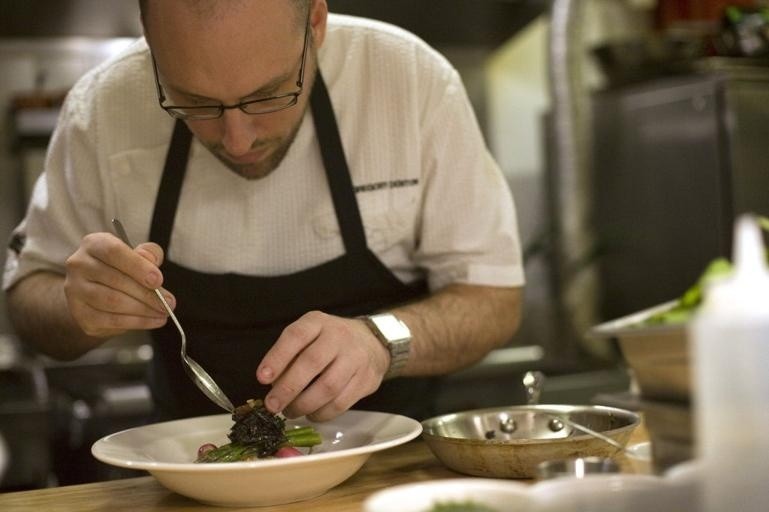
[1,3,527,433]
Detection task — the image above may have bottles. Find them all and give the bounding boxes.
[687,211,768,512]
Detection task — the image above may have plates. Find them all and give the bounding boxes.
[91,407,423,509]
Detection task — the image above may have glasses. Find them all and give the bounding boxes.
[149,4,310,122]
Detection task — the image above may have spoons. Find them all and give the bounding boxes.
[113,216,241,415]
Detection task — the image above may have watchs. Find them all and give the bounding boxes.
[357,311,412,382]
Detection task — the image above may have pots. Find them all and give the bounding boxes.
[420,370,640,479]
